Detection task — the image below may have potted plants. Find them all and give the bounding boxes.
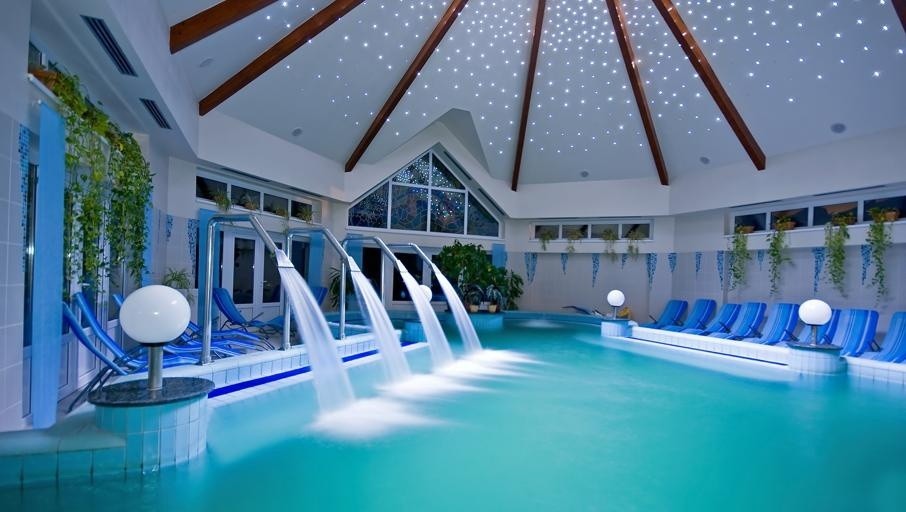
[27,56,157,293]
[727,215,796,296]
[824,204,898,297]
[534,226,647,267]
[437,239,525,314]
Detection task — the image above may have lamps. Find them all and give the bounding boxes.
[606,285,626,320]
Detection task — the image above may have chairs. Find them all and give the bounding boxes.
[638,297,906,367]
[561,303,632,319]
[57,283,328,412]
